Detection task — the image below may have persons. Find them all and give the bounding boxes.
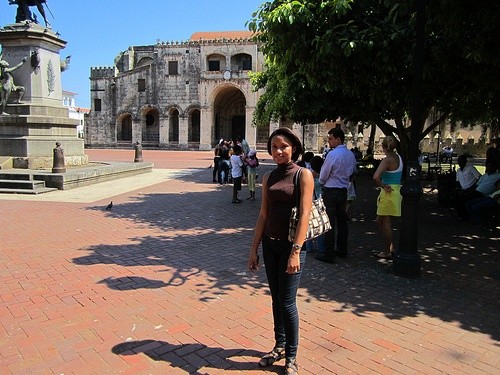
[230,146,244,203]
[212,134,249,185]
[250,128,315,375]
[0,57,27,116]
[373,136,404,259]
[315,127,357,263]
[444,147,454,164]
[295,148,334,253]
[363,148,373,163]
[456,148,500,230]
[243,149,259,201]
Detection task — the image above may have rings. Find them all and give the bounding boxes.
[294,269,297,272]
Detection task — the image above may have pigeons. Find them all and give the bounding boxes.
[66,54,73,60]
[208,164,212,168]
[0,22,61,36]
[105,200,113,211]
[136,140,141,145]
[31,50,40,55]
[55,141,62,148]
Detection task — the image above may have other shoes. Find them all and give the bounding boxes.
[213,179,233,184]
[247,196,252,199]
[251,197,255,200]
[374,252,393,259]
[232,198,242,203]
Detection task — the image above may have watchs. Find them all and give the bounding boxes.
[292,244,302,250]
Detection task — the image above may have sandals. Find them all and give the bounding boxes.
[258,346,286,368]
[284,357,299,375]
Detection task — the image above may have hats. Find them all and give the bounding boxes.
[248,150,257,157]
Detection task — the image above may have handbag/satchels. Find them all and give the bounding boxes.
[287,169,332,244]
[346,181,356,200]
